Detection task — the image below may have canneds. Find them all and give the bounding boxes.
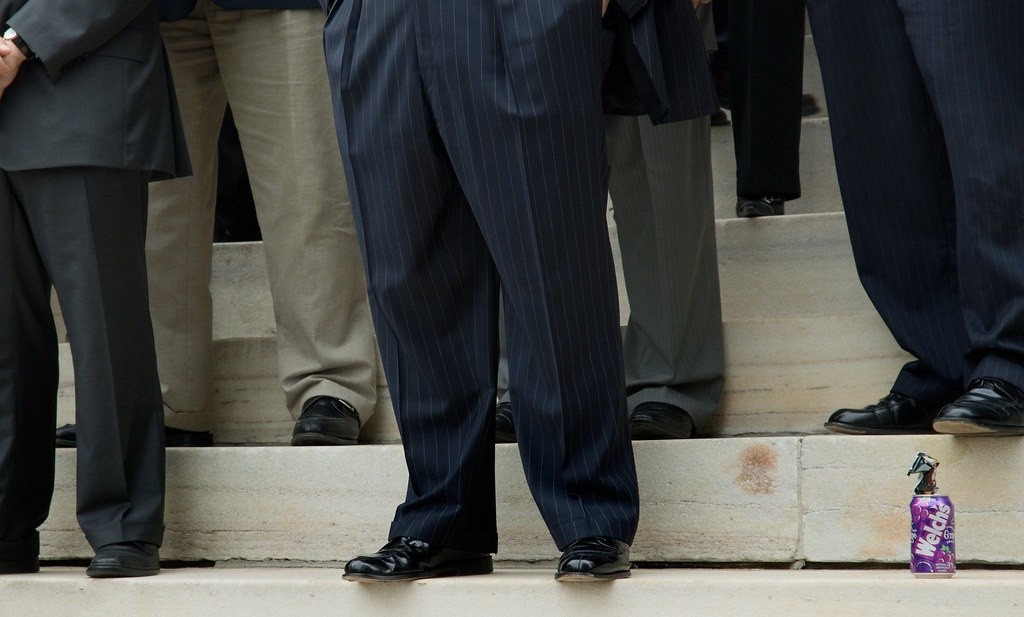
[908,495,956,579]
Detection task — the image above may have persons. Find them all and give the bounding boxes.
[318,0,724,581]
[56,0,376,448]
[0,0,193,577]
[808,0,1024,438]
[696,0,824,218]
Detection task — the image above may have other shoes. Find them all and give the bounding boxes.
[709,109,731,126]
[212,216,262,242]
[736,198,785,216]
[801,95,820,115]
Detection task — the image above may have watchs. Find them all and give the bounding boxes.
[3,26,35,60]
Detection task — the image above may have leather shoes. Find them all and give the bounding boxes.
[555,536,632,580]
[630,402,693,438]
[0,557,39,574]
[342,536,493,582]
[291,396,360,445]
[933,377,1024,435]
[494,402,517,442]
[824,392,938,434]
[86,543,160,577]
[55,423,214,447]
